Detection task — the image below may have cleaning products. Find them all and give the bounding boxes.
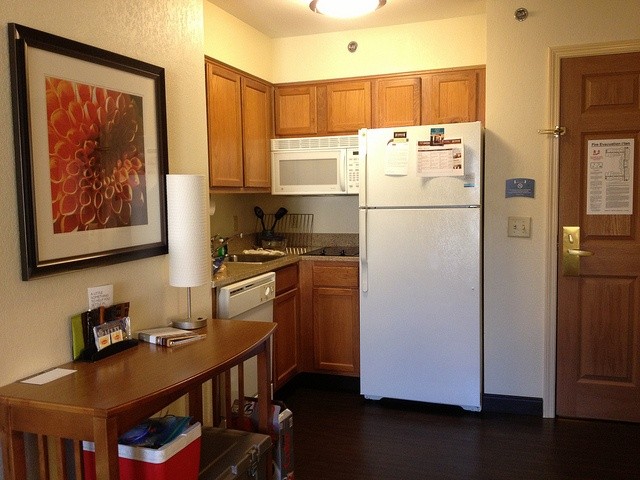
[217,239,224,256]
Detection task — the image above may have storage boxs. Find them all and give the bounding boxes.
[228,396,294,480]
[81,422,204,479]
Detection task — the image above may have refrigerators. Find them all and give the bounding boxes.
[358,121,482,412]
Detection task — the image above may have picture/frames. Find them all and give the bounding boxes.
[5,20,170,284]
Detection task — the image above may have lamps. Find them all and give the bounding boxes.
[309,0,387,23]
[163,171,210,330]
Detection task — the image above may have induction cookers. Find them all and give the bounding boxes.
[300,246,359,256]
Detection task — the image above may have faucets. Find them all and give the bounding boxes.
[212,232,246,258]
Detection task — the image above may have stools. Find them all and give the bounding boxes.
[197,424,274,480]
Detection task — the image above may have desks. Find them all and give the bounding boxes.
[1,317,281,480]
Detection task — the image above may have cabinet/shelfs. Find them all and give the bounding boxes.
[423,65,486,126]
[323,76,371,137]
[310,261,361,377]
[272,258,302,391]
[205,55,270,194]
[371,72,422,129]
[271,80,317,138]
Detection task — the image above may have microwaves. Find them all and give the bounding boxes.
[269,135,358,195]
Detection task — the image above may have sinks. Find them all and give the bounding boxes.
[225,253,285,265]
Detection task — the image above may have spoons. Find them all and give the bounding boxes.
[254,206,266,231]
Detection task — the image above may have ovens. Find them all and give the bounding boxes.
[218,272,276,414]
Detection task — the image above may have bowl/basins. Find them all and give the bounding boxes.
[255,238,288,249]
